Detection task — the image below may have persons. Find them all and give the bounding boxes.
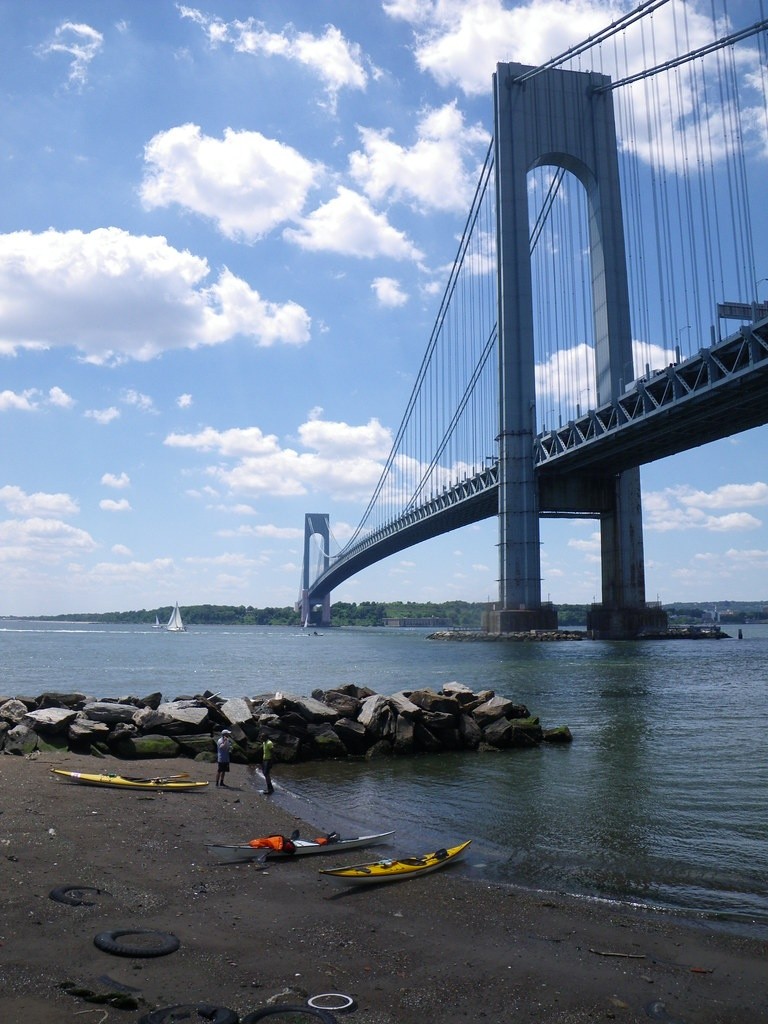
[261,733,275,795]
[214,730,232,786]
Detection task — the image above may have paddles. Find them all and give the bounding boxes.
[134,773,189,782]
[253,829,300,865]
[424,848,448,864]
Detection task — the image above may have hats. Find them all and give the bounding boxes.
[222,730,232,735]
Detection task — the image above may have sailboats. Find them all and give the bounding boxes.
[152,615,163,629]
[165,601,186,632]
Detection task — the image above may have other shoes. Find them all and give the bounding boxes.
[215,785,220,787]
[263,790,275,795]
[220,784,227,786]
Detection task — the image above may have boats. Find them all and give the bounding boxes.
[49,767,210,791]
[308,632,324,636]
[318,839,474,884]
[204,830,397,859]
